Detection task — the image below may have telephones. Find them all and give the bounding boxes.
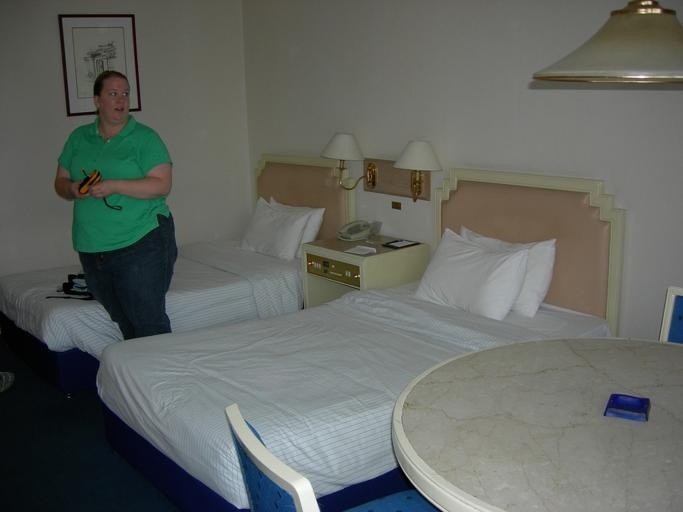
[336,220,372,239]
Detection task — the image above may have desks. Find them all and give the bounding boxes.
[389,335,683,511]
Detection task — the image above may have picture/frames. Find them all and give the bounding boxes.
[56,12,142,117]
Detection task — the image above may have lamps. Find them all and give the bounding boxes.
[527,0,683,91]
[319,132,378,191]
[393,135,442,202]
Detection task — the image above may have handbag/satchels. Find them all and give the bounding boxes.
[63,274,90,295]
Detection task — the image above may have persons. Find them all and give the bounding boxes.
[54,70,178,342]
